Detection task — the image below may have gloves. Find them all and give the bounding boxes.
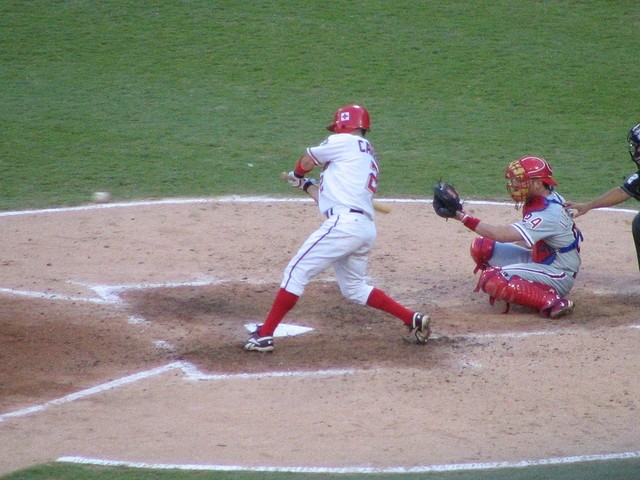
[300,177,316,191]
[288,171,303,188]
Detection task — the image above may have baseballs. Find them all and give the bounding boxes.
[93,191,111,203]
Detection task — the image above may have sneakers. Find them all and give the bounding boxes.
[410,311,431,343]
[244,324,275,353]
[550,299,575,318]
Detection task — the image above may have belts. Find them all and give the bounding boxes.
[326,207,364,219]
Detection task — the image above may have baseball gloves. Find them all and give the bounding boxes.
[433,180,464,219]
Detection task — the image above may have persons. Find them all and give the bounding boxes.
[245,105,431,352]
[453,156,582,318]
[561,122,640,270]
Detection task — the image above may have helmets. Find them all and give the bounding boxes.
[626,125,640,169]
[327,105,370,133]
[505,156,557,211]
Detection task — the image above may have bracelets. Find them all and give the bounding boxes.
[463,215,481,232]
[295,155,312,175]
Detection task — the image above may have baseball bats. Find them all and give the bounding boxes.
[281,172,390,213]
